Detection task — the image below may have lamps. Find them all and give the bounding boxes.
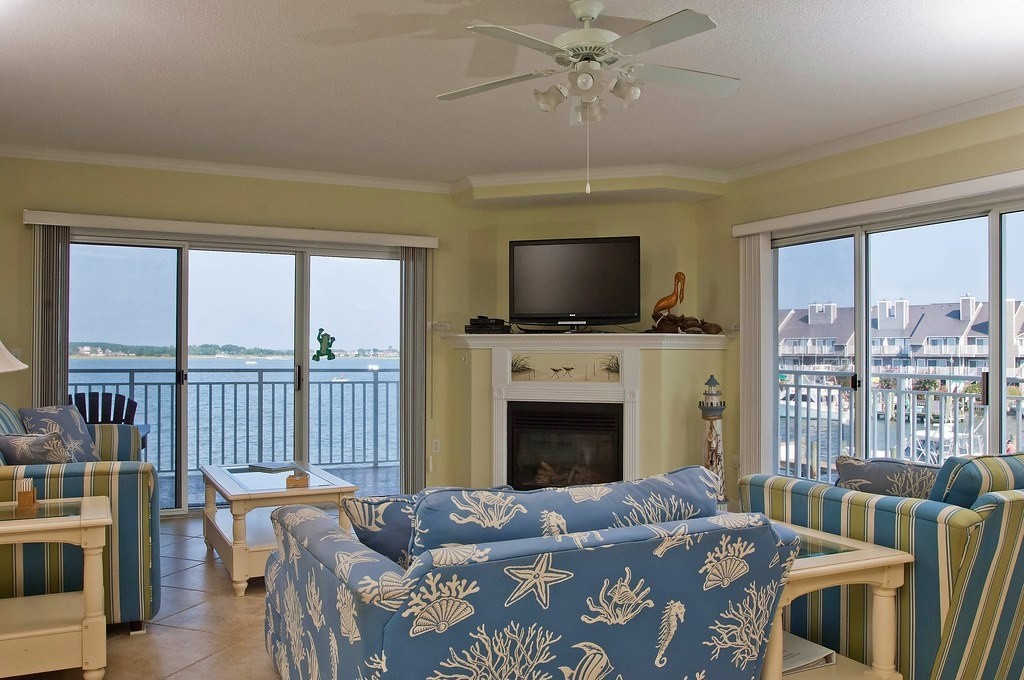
[532,61,645,198]
[0,339,29,374]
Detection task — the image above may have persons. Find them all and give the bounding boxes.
[1006,440,1014,455]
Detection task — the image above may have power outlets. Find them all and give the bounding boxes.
[433,438,440,453]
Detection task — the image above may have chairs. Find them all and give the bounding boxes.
[68,392,151,462]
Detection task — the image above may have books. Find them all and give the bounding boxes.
[250,463,295,472]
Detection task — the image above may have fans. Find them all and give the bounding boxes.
[436,0,740,100]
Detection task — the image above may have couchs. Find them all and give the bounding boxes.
[263,465,801,680]
[736,451,1024,680]
[0,401,161,631]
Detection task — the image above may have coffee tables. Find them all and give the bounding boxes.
[198,462,358,596]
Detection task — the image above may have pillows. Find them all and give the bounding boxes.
[341,484,514,569]
[18,403,102,462]
[834,455,942,501]
[0,432,78,466]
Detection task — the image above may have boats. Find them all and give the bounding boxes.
[331,375,351,382]
[844,415,985,465]
[780,440,837,475]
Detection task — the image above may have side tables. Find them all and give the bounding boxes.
[758,519,914,680]
[0,496,113,680]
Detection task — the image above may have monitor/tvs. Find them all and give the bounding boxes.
[510,236,641,334]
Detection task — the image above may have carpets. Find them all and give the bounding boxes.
[159,464,401,509]
